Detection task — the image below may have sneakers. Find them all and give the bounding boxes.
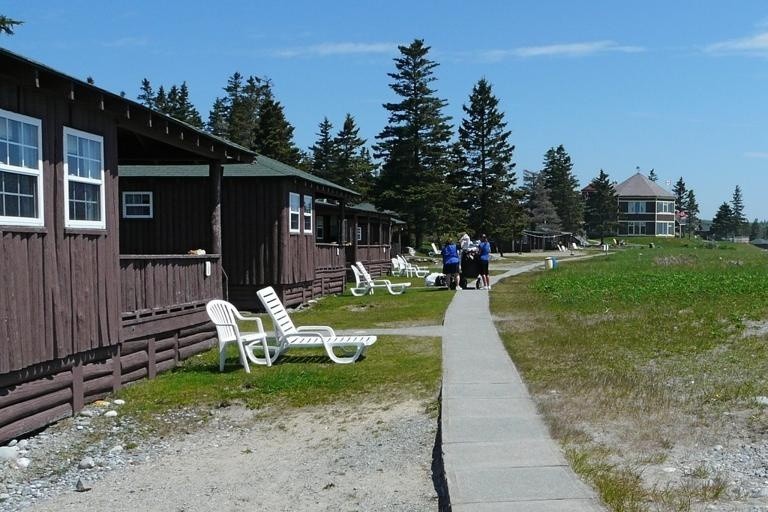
[456,286,463,290]
[480,287,488,290]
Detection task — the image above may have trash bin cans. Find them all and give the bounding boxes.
[544,257,553,269]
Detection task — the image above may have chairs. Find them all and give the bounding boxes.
[557,242,583,253]
[244,286,377,365]
[350,243,443,297]
[206,300,271,373]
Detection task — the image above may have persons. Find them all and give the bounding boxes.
[478,233,491,289]
[457,229,471,273]
[441,238,463,291]
